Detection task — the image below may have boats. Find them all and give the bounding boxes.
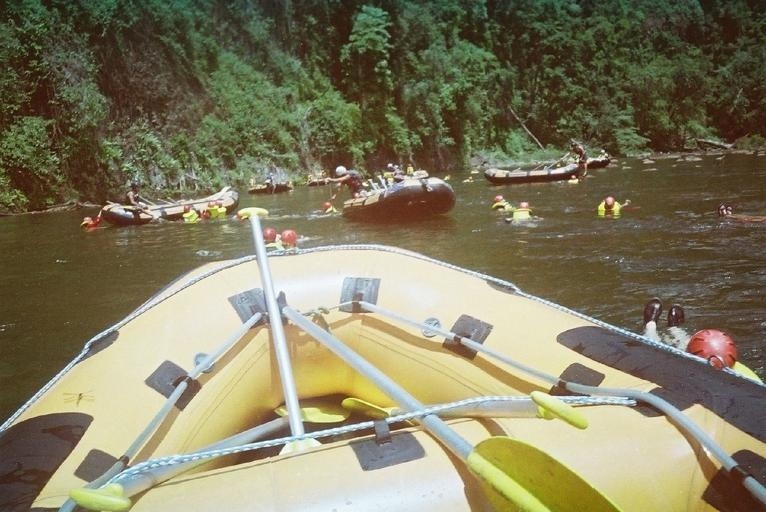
[1,243,761,512]
[347,169,457,219]
[104,187,239,226]
[482,146,609,183]
[695,138,732,150]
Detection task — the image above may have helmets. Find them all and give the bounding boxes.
[83,216,92,221]
[520,201,529,208]
[130,182,139,187]
[336,165,347,176]
[494,195,503,201]
[687,328,736,369]
[605,197,614,208]
[263,227,297,242]
[322,201,331,209]
[184,200,222,219]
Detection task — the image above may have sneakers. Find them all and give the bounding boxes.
[668,305,684,327]
[718,203,733,217]
[644,297,663,328]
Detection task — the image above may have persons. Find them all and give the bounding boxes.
[491,195,512,211]
[718,203,766,222]
[568,175,580,185]
[568,139,594,178]
[141,208,174,227]
[125,183,142,207]
[512,200,532,219]
[322,201,338,214]
[387,161,415,182]
[596,197,632,219]
[247,172,294,189]
[218,203,226,218]
[180,205,197,225]
[641,296,763,392]
[323,164,370,202]
[265,225,280,244]
[278,229,297,250]
[202,202,219,218]
[81,210,105,231]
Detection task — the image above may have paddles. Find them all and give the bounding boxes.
[274,291,624,512]
